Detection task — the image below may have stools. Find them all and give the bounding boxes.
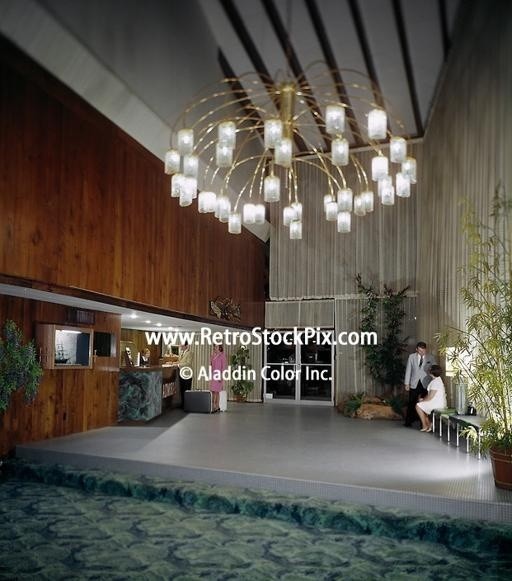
[184,389,228,412]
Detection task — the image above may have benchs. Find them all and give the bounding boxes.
[430,407,490,460]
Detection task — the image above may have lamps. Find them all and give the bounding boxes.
[445,346,475,414]
[162,1,422,239]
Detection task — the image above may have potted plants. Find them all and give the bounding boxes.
[436,180,512,492]
[230,346,253,403]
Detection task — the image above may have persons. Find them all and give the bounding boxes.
[403,342,447,432]
[208,341,226,414]
[177,339,194,408]
[140,347,151,366]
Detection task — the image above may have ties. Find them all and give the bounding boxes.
[419,355,423,367]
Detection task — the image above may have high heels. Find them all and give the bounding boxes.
[420,422,433,433]
[211,408,220,414]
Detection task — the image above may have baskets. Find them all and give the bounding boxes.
[163,356,179,362]
